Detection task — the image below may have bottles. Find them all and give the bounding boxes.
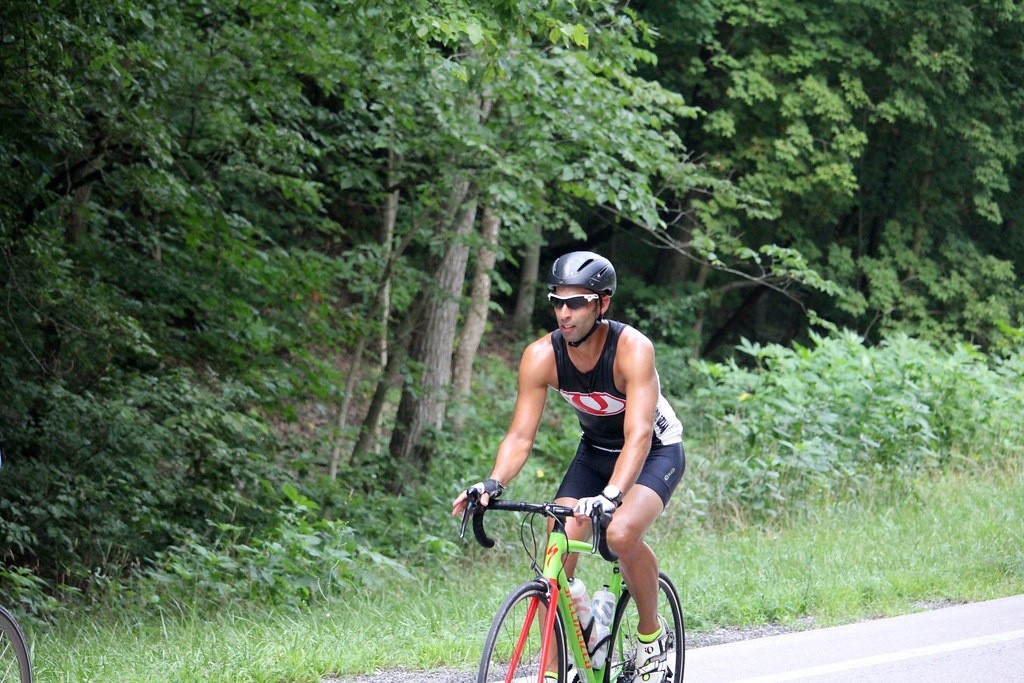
[568,577,597,650]
[587,584,616,670]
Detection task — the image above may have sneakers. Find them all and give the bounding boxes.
[544,664,581,683]
[633,614,669,683]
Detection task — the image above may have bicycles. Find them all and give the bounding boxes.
[460,485,686,683]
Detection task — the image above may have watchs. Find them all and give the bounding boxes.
[601,485,622,507]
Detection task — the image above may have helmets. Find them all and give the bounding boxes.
[548,251,617,297]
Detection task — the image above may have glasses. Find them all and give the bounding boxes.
[547,292,599,310]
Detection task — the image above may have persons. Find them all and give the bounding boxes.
[452,251,686,682]
